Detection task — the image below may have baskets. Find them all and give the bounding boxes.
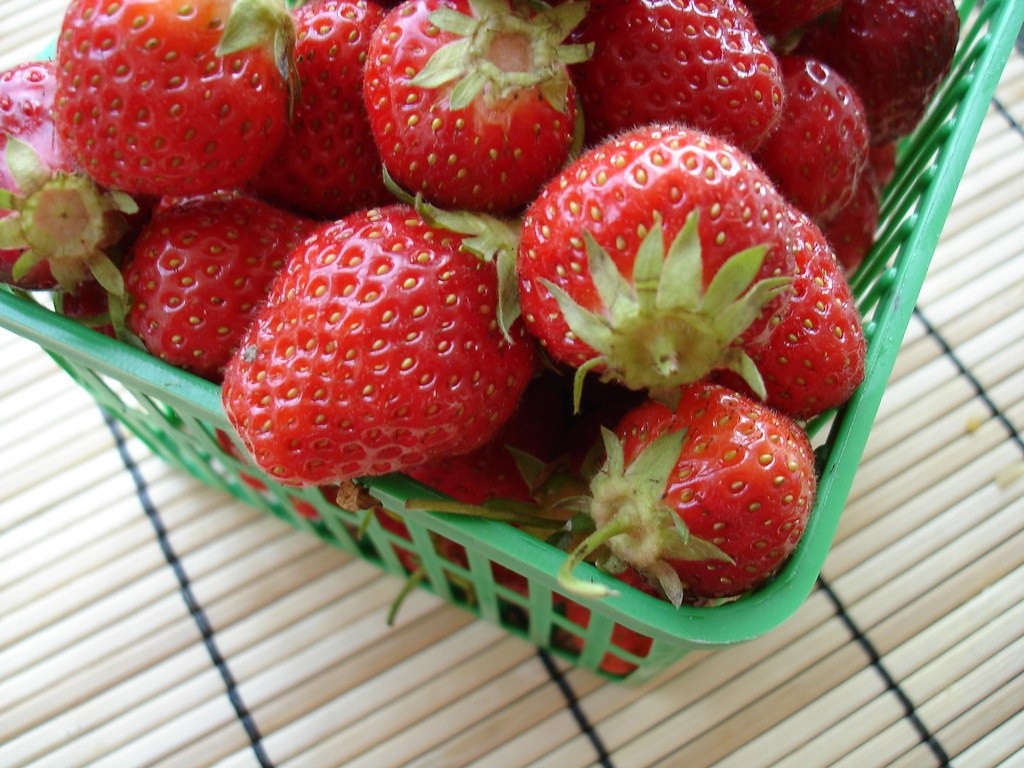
[0,0,1024,683]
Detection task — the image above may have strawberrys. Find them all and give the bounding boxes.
[0,1,960,675]
